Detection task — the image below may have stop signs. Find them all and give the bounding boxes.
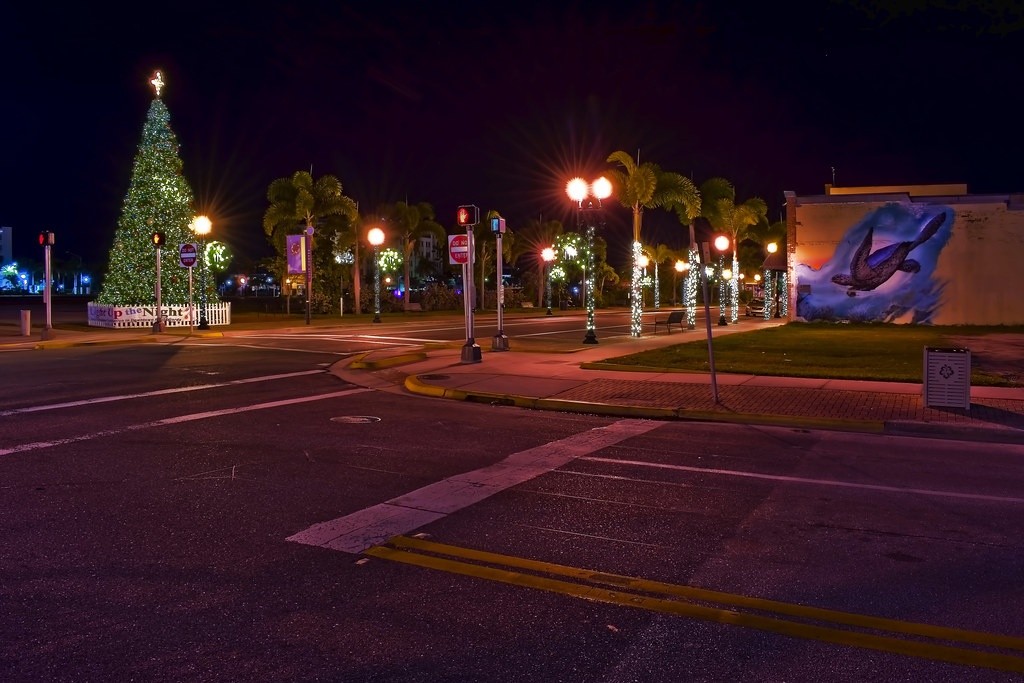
[178,243,196,266]
[449,236,475,264]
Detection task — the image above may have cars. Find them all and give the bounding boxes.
[746,297,777,317]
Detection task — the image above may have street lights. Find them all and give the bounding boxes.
[567,177,614,344]
[194,216,211,330]
[766,243,781,318]
[716,236,729,325]
[674,261,684,303]
[367,228,385,323]
[541,249,555,315]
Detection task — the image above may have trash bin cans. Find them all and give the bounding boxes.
[922,344,971,412]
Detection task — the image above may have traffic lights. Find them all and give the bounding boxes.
[39,232,54,245]
[490,219,506,234]
[153,232,165,245]
[456,204,477,227]
